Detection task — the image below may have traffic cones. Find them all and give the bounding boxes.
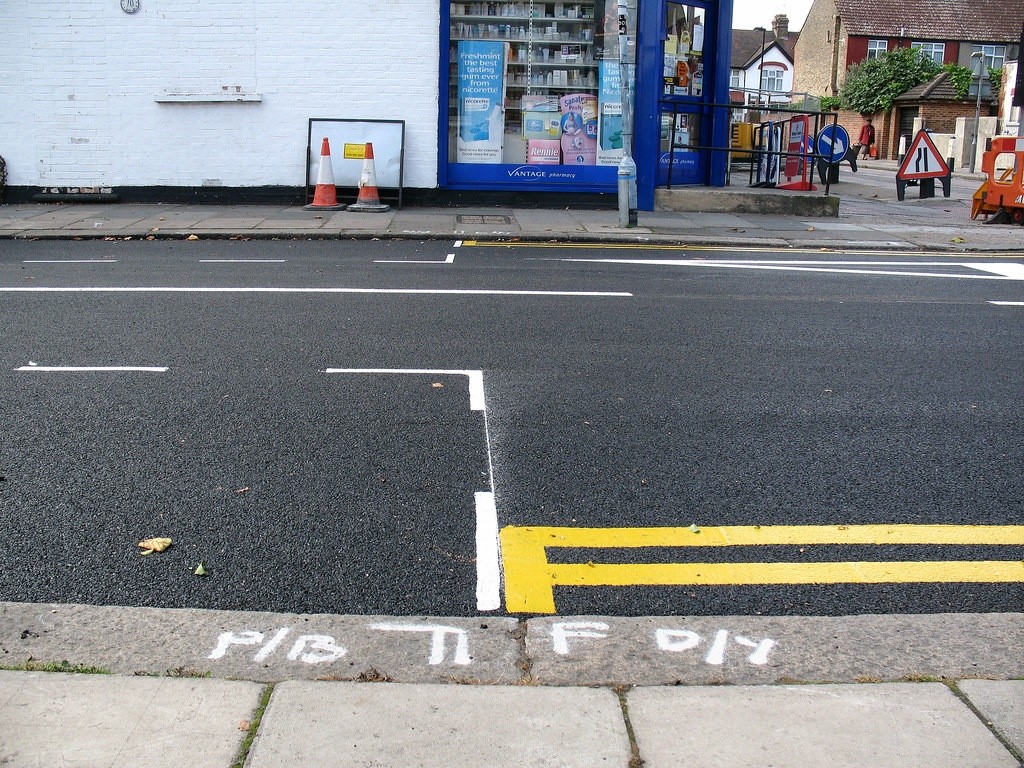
[301,138,348,211]
[345,143,391,213]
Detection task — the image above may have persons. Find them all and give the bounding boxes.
[857,119,875,160]
[677,136,682,144]
[692,73,702,85]
[575,137,584,148]
[577,157,582,163]
[682,32,689,43]
[563,112,581,136]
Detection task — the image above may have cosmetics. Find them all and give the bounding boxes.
[451,3,596,100]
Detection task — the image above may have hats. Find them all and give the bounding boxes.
[866,118,872,121]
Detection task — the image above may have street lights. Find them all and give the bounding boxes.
[969,52,985,173]
[753,26,766,107]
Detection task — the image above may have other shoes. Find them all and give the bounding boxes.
[863,157,867,160]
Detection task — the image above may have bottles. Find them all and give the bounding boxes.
[538,70,543,86]
[517,45,526,63]
[587,69,596,87]
[516,72,527,84]
[519,26,525,40]
[536,47,543,62]
[505,24,511,39]
[469,1,501,16]
[506,93,522,108]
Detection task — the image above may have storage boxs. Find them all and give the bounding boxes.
[522,95,562,164]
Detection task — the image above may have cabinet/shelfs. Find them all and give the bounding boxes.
[450,0,594,133]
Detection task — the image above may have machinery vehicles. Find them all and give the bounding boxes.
[970,136,1024,226]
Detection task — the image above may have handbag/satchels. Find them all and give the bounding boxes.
[869,144,878,156]
